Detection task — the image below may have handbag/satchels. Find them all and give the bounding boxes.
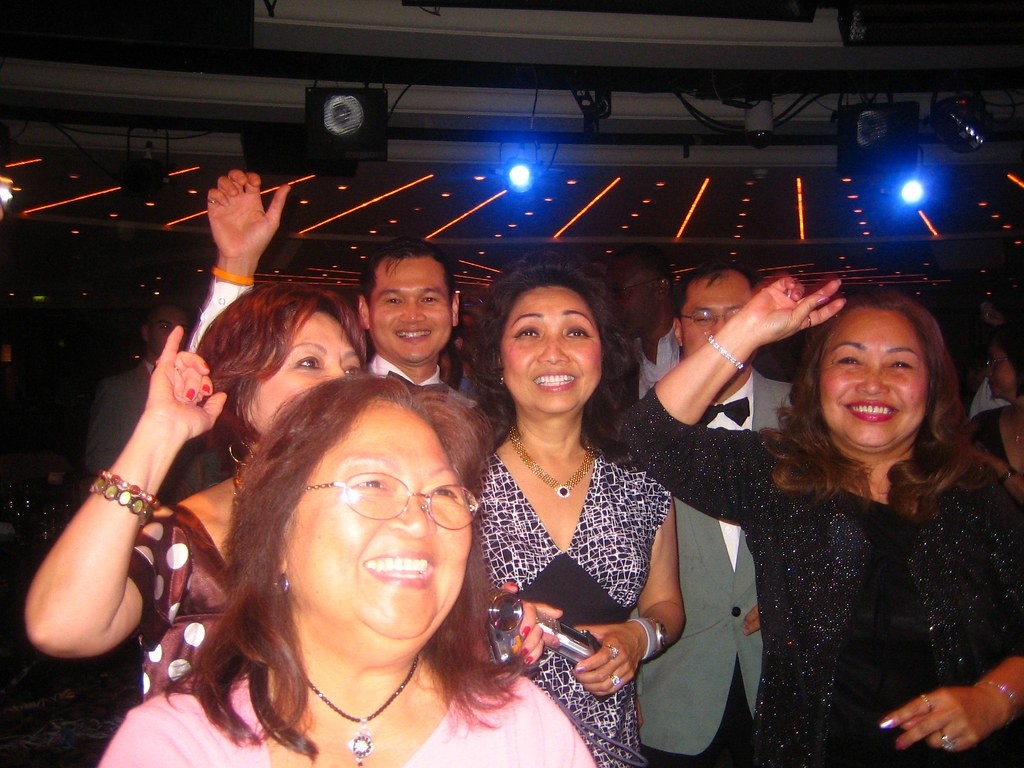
[519,553,631,652]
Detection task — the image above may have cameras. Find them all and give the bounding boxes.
[535,611,594,664]
[484,589,524,664]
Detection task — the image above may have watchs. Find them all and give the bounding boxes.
[643,616,668,656]
[998,466,1017,483]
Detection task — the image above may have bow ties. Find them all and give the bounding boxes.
[386,371,449,402]
[700,397,750,427]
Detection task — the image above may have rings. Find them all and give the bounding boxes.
[938,730,956,752]
[920,694,933,712]
[175,365,181,375]
[207,198,217,204]
[785,292,797,302]
[604,644,618,659]
[806,315,811,327]
[609,675,620,687]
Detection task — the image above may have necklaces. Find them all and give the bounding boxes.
[859,487,888,497]
[305,654,418,767]
[510,424,596,497]
[1009,412,1024,444]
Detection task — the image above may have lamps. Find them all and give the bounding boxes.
[928,86,995,154]
[833,98,922,179]
[306,81,391,164]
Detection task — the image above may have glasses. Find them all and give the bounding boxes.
[680,308,740,326]
[987,357,1008,369]
[617,278,672,305]
[306,472,481,530]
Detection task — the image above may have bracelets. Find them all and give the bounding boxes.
[627,618,655,659]
[975,681,1017,727]
[707,335,743,370]
[210,266,254,286]
[99,470,161,508]
[90,477,152,524]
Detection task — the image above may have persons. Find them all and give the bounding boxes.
[24,169,1024,768]
